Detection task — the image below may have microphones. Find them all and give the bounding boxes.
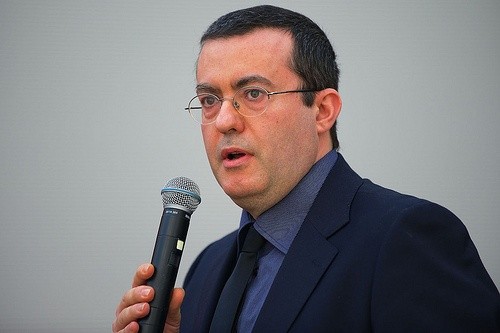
[137,177,202,333]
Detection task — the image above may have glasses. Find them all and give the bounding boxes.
[185,85,318,126]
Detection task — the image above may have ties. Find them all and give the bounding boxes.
[207,224,264,333]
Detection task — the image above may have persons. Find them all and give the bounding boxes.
[113,6,499,333]
